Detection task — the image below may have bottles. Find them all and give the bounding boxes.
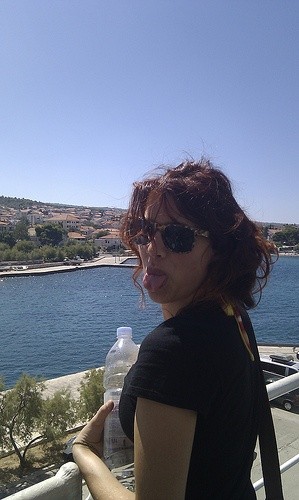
[102,327,139,492]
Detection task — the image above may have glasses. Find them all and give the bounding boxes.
[128,218,211,254]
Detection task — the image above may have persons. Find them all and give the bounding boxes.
[72,151,285,500]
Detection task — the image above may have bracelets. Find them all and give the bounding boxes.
[73,441,100,455]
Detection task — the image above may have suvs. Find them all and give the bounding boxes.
[259,353,299,412]
